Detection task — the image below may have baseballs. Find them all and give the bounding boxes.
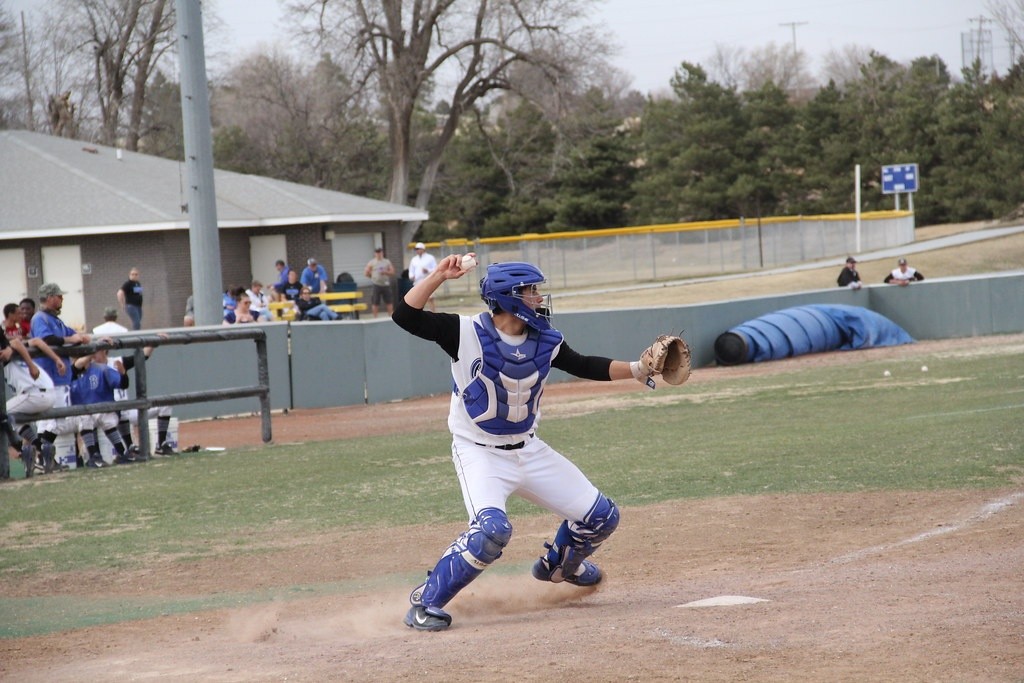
[460,255,477,272]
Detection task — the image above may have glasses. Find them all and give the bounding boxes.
[244,300,252,305]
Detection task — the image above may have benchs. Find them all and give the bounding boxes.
[270,292,365,320]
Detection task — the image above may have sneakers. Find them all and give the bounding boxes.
[40,443,56,475]
[113,453,126,463]
[532,556,602,586]
[404,605,448,632]
[86,451,104,468]
[20,445,36,478]
[125,446,146,462]
[155,441,173,455]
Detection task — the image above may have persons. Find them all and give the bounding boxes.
[185,241,343,324]
[837,257,861,290]
[391,252,690,632]
[408,242,437,312]
[884,259,924,286]
[0,284,181,478]
[117,268,143,330]
[365,248,394,317]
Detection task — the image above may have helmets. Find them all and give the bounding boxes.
[479,263,554,329]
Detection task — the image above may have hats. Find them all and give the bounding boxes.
[414,242,426,250]
[37,282,69,298]
[375,247,383,253]
[846,257,856,263]
[103,306,118,317]
[898,259,906,266]
[307,258,318,265]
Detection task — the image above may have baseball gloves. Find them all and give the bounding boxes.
[640,336,692,385]
